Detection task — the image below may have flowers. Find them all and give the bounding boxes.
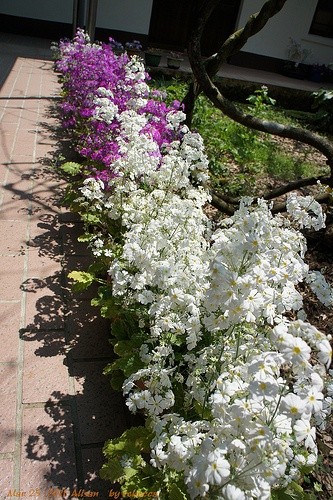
[125,40,143,51]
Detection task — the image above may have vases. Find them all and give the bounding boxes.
[128,50,139,58]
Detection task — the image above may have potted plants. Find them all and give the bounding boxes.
[310,63,326,83]
[166,50,185,69]
[143,47,162,67]
